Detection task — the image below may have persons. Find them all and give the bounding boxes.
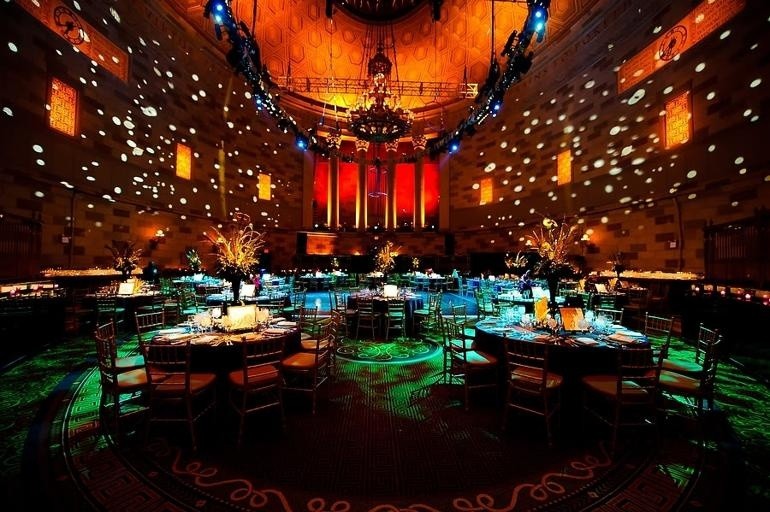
[519,270,539,314]
[251,272,262,296]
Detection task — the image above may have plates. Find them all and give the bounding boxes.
[482,310,629,346]
[160,313,296,346]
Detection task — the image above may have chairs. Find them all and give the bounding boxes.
[292,268,478,350]
[440,312,501,411]
[474,262,770,377]
[660,335,722,453]
[300,309,342,385]
[229,335,285,448]
[502,343,565,445]
[1,272,307,354]
[584,344,666,457]
[139,340,217,453]
[94,332,175,450]
[282,318,330,415]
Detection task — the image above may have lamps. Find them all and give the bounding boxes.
[322,0,429,162]
